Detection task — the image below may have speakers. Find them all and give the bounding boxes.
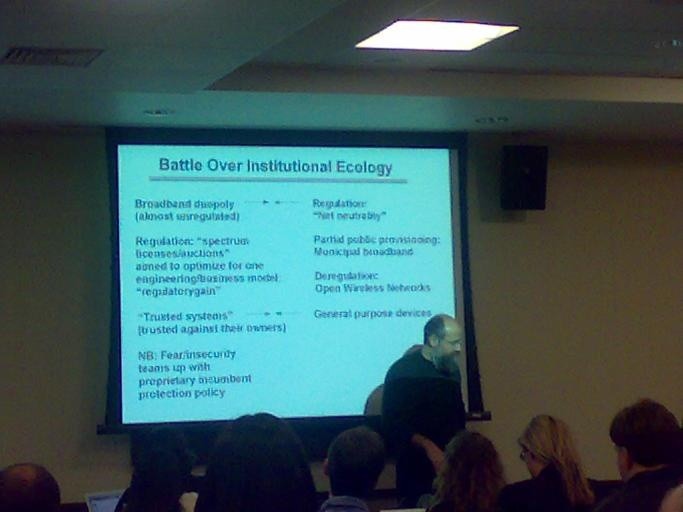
[502,144,549,212]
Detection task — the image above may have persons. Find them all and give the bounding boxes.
[194,413,319,510]
[114,451,198,511]
[417,429,505,511]
[381,314,467,507]
[592,399,683,512]
[497,414,600,511]
[318,429,387,511]
[0,461,60,512]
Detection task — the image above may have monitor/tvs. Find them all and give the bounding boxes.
[83,487,127,512]
